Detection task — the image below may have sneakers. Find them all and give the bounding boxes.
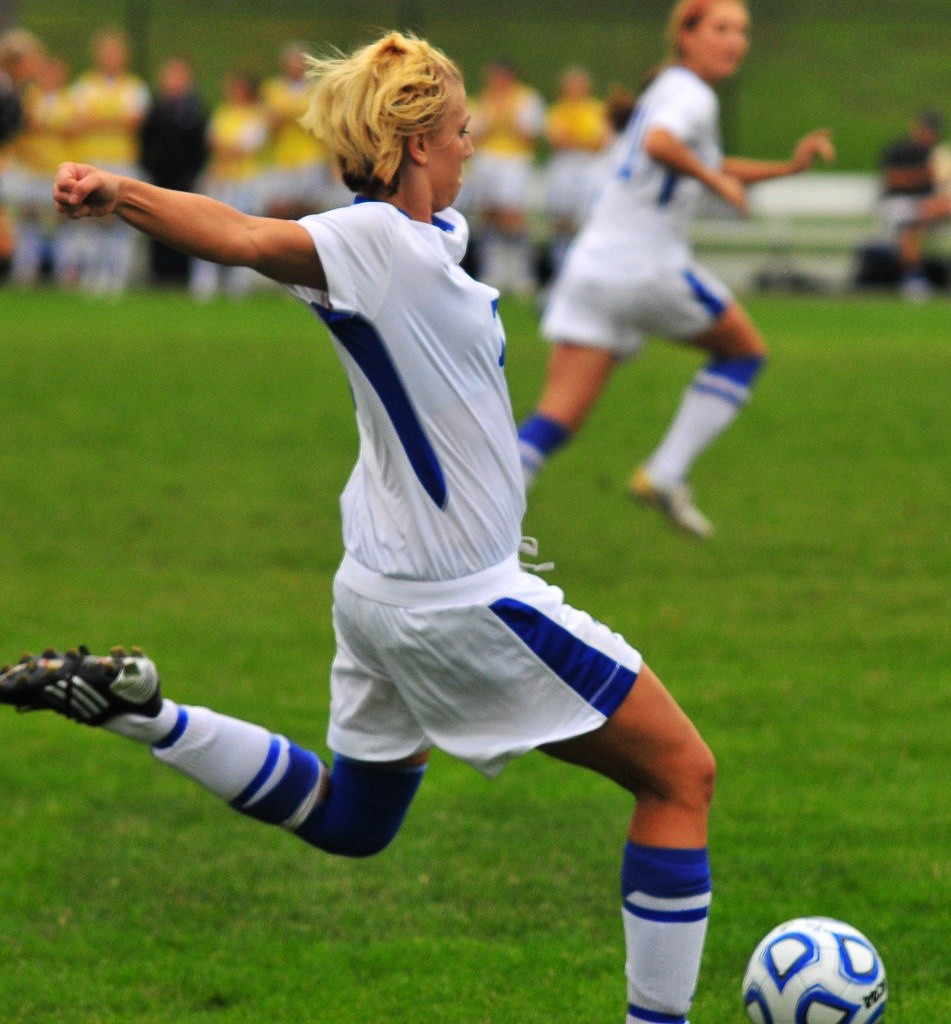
[630,467,714,540]
[0,645,164,727]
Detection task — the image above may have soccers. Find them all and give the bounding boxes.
[740,913,891,1024]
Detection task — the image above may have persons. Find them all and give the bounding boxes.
[849,109,950,294]
[0,25,657,298]
[514,0,833,535]
[0,28,717,1024]
